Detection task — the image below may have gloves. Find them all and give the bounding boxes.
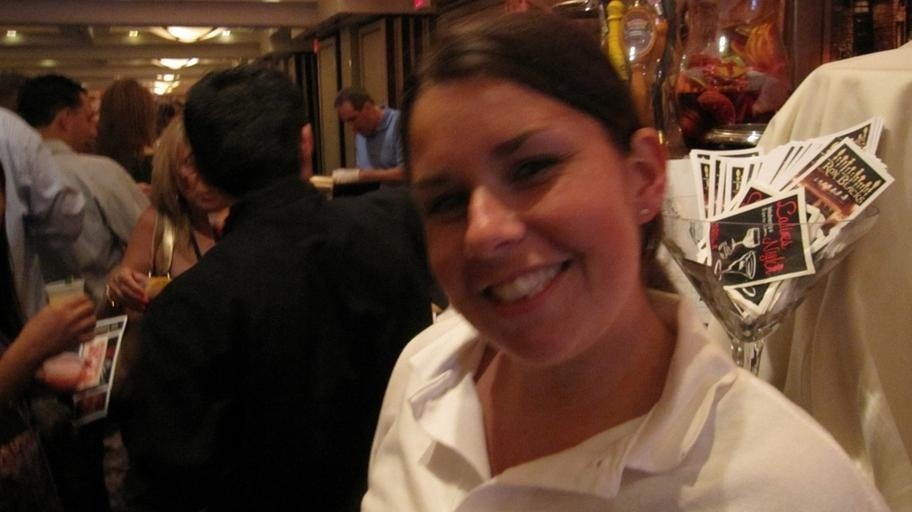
[333,167,361,185]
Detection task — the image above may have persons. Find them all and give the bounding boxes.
[121,60,436,509]
[0,105,89,423]
[104,115,232,307]
[731,34,912,512]
[1,291,100,511]
[0,73,191,280]
[323,83,412,190]
[354,8,890,512]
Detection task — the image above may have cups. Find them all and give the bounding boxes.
[46,280,84,308]
[141,271,171,301]
[45,355,83,387]
